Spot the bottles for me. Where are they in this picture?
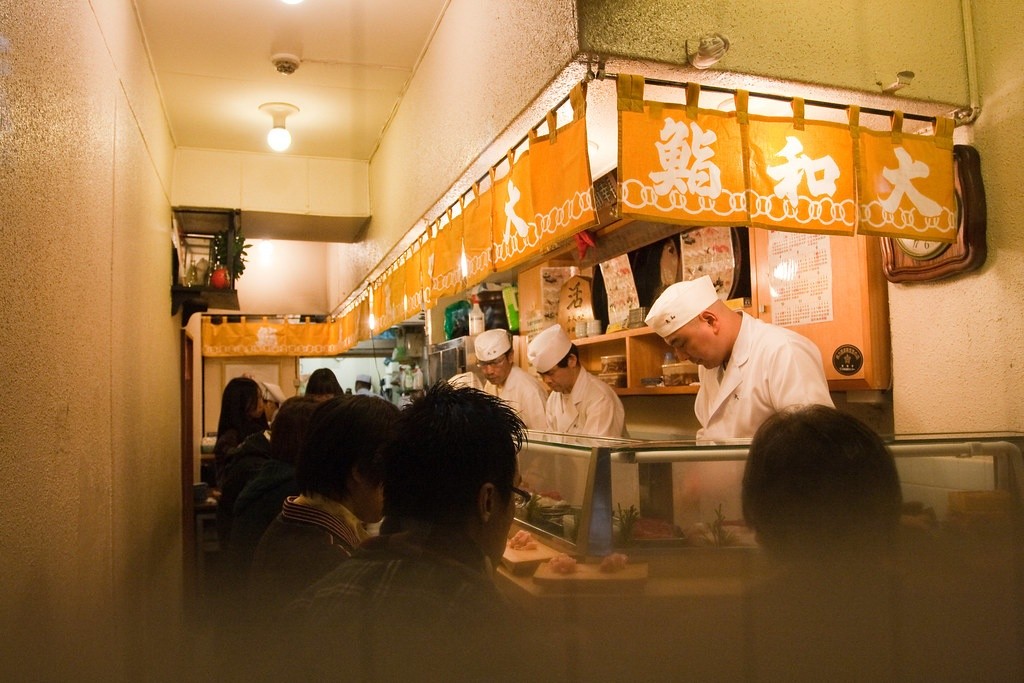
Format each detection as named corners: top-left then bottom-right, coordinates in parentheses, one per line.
top-left (664, 352), bottom-right (675, 364)
top-left (469, 299), bottom-right (485, 335)
top-left (400, 365), bottom-right (423, 389)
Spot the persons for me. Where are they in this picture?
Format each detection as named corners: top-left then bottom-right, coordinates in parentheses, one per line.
top-left (528, 326), bottom-right (638, 515)
top-left (470, 326), bottom-right (546, 430)
top-left (723, 405), bottom-right (905, 550)
top-left (644, 281), bottom-right (832, 536)
top-left (206, 369), bottom-right (530, 637)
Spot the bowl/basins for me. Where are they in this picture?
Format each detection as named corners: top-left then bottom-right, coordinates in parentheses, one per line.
top-left (575, 320), bottom-right (602, 338)
top-left (597, 373), bottom-right (627, 388)
top-left (600, 355), bottom-right (627, 373)
top-left (661, 363), bottom-right (699, 386)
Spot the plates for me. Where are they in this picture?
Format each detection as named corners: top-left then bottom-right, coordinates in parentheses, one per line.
top-left (627, 526), bottom-right (689, 542)
top-left (533, 562), bottom-right (649, 582)
top-left (501, 539), bottom-right (559, 573)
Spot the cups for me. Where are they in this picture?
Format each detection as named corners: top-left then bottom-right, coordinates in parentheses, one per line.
top-left (193, 482), bottom-right (208, 504)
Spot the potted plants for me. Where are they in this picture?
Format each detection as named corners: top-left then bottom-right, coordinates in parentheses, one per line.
top-left (209, 227), bottom-right (251, 290)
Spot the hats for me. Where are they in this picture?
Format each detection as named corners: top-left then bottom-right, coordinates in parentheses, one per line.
top-left (474, 329), bottom-right (511, 363)
top-left (356, 374), bottom-right (372, 383)
top-left (527, 323), bottom-right (572, 374)
top-left (644, 273), bottom-right (717, 337)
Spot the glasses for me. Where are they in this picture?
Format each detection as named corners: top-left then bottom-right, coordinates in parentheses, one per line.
top-left (474, 354), bottom-right (507, 368)
top-left (481, 480), bottom-right (532, 508)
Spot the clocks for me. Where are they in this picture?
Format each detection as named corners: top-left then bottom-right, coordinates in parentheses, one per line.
top-left (893, 186), bottom-right (962, 262)
